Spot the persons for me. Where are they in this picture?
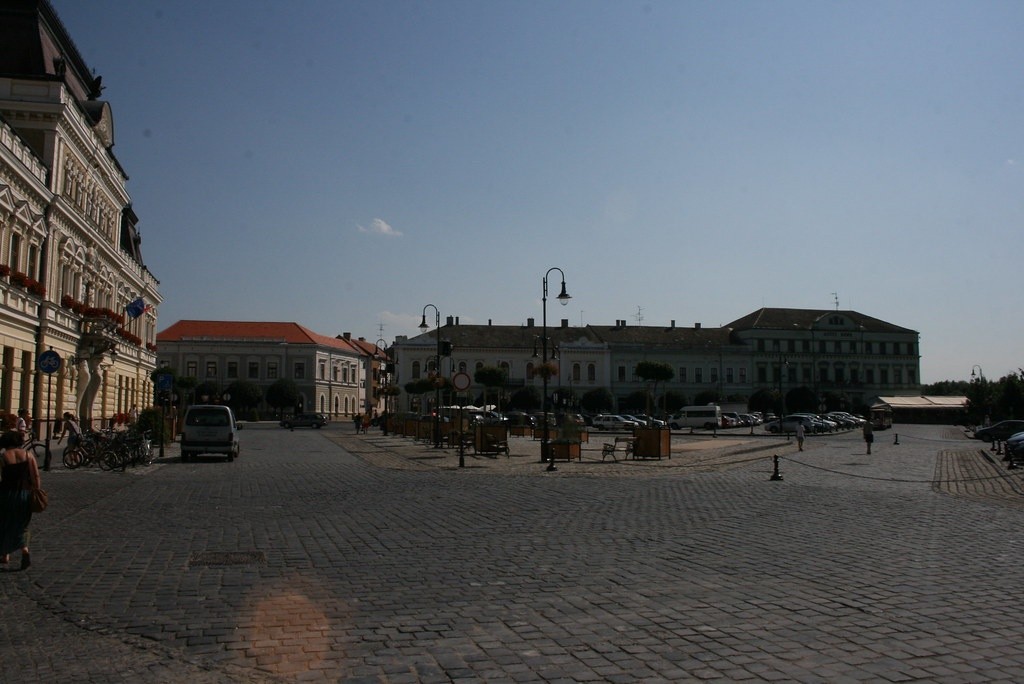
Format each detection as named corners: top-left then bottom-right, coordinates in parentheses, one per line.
top-left (362, 412), bottom-right (369, 434)
top-left (129, 404), bottom-right (138, 424)
top-left (863, 417), bottom-right (873, 454)
top-left (985, 415), bottom-right (990, 427)
top-left (0, 409), bottom-right (32, 453)
top-left (796, 421), bottom-right (805, 451)
top-left (374, 410), bottom-right (378, 419)
top-left (0, 430), bottom-right (40, 565)
top-left (57, 412), bottom-right (80, 466)
top-left (354, 413), bottom-right (361, 434)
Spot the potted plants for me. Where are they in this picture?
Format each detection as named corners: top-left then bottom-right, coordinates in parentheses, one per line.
top-left (630, 359), bottom-right (676, 462)
top-left (382, 365), bottom-right (590, 462)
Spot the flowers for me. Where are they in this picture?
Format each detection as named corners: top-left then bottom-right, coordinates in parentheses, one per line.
top-left (62, 294), bottom-right (159, 352)
top-left (0, 262), bottom-right (45, 295)
top-left (112, 412), bottom-right (131, 425)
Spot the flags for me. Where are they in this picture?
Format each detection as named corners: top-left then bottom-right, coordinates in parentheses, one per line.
top-left (126, 299), bottom-right (144, 318)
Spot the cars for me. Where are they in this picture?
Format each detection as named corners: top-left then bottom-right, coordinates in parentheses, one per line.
top-left (974, 420), bottom-right (1024, 461)
top-left (429, 403), bottom-right (874, 435)
top-left (280, 411), bottom-right (328, 429)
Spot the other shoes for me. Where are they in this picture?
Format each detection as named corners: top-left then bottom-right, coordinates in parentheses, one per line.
top-left (21, 551), bottom-right (31, 570)
top-left (800, 448), bottom-right (803, 451)
top-left (867, 450), bottom-right (871, 454)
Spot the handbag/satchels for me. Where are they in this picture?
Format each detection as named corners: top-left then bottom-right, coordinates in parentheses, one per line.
top-left (24, 486), bottom-right (48, 514)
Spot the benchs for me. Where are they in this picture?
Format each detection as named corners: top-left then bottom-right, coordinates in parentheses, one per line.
top-left (600, 434), bottom-right (641, 462)
top-left (484, 430), bottom-right (511, 460)
top-left (454, 430), bottom-right (474, 451)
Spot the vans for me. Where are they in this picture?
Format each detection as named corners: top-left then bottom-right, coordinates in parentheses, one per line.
top-left (667, 405), bottom-right (723, 430)
top-left (180, 404), bottom-right (243, 463)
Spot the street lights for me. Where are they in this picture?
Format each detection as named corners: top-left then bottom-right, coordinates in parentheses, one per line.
top-left (971, 364), bottom-right (986, 427)
top-left (373, 339), bottom-right (399, 437)
top-left (532, 267), bottom-right (573, 472)
top-left (793, 323), bottom-right (818, 413)
top-left (417, 304), bottom-right (456, 452)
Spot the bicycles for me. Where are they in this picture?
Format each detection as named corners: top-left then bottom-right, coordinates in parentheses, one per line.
top-left (17, 424), bottom-right (155, 472)
